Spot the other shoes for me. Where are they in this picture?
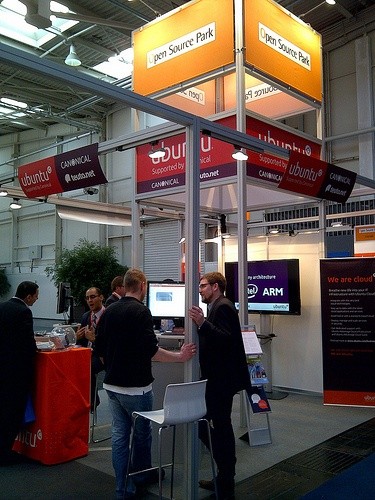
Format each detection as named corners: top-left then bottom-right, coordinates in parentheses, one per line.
top-left (91, 399), bottom-right (100, 411)
top-left (203, 493), bottom-right (218, 500)
top-left (130, 487), bottom-right (148, 500)
top-left (199, 479), bottom-right (215, 491)
top-left (0, 451), bottom-right (29, 465)
top-left (136, 469), bottom-right (165, 487)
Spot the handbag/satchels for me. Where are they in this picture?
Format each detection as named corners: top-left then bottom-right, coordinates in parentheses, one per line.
top-left (23, 395), bottom-right (35, 426)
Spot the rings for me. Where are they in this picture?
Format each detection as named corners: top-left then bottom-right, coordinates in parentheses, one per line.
top-left (192, 350), bottom-right (194, 353)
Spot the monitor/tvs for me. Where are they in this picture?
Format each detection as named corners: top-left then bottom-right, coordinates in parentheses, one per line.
top-left (147, 281), bottom-right (209, 318)
top-left (223, 260), bottom-right (301, 315)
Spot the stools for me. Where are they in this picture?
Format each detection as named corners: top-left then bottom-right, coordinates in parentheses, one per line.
top-left (122, 379), bottom-right (218, 500)
top-left (92, 373), bottom-right (111, 444)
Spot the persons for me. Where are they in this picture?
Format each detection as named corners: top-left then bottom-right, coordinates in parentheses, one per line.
top-left (94, 268), bottom-right (198, 500)
top-left (0, 281), bottom-right (38, 461)
top-left (75, 287), bottom-right (107, 411)
top-left (189, 272), bottom-right (252, 500)
top-left (106, 276), bottom-right (126, 308)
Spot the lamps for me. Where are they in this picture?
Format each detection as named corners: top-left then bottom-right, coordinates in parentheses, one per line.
top-left (10, 197), bottom-right (22, 209)
top-left (232, 143), bottom-right (249, 161)
top-left (65, 43), bottom-right (82, 67)
top-left (148, 141), bottom-right (166, 158)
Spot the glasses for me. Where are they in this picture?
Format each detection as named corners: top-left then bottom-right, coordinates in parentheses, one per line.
top-left (199, 282), bottom-right (221, 288)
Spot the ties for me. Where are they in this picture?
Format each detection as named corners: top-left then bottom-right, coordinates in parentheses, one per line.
top-left (91, 314), bottom-right (97, 329)
